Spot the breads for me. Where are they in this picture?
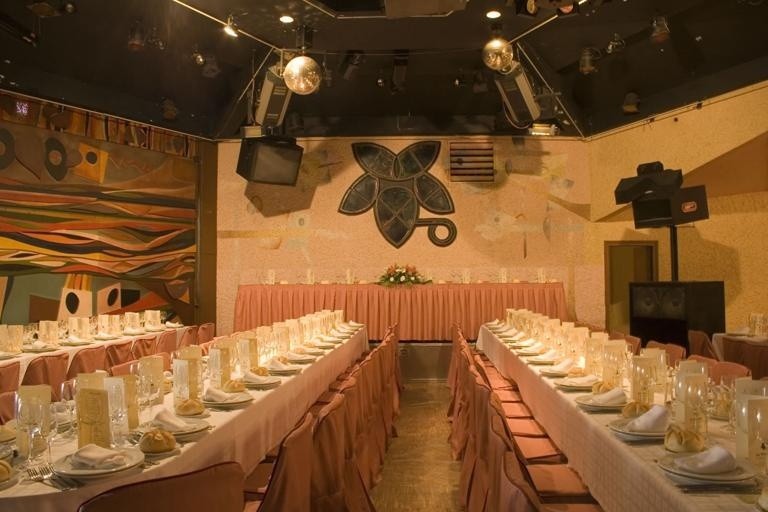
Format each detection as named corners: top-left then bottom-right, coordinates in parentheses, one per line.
top-left (304, 342), bottom-right (316, 348)
top-left (277, 356), bottom-right (288, 365)
top-left (1, 460), bottom-right (12, 482)
top-left (518, 335), bottom-right (704, 453)
top-left (294, 347), bottom-right (306, 355)
top-left (140, 428), bottom-right (176, 454)
top-left (223, 380), bottom-right (245, 392)
top-left (175, 397), bottom-right (204, 415)
top-left (250, 366), bottom-right (269, 376)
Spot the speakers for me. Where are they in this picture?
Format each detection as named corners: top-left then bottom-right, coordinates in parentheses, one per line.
top-left (629, 281), bottom-right (726, 358)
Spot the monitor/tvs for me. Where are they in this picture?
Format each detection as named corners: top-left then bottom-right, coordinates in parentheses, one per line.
top-left (236, 137), bottom-right (304, 186)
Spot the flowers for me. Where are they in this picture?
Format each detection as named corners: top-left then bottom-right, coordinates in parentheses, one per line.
top-left (375, 264), bottom-right (432, 283)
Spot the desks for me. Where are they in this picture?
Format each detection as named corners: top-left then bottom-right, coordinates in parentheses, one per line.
top-left (234, 283), bottom-right (568, 342)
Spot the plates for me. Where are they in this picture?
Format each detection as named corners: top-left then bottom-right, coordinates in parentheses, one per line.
top-left (0, 322), bottom-right (364, 493)
top-left (481, 319), bottom-right (756, 509)
